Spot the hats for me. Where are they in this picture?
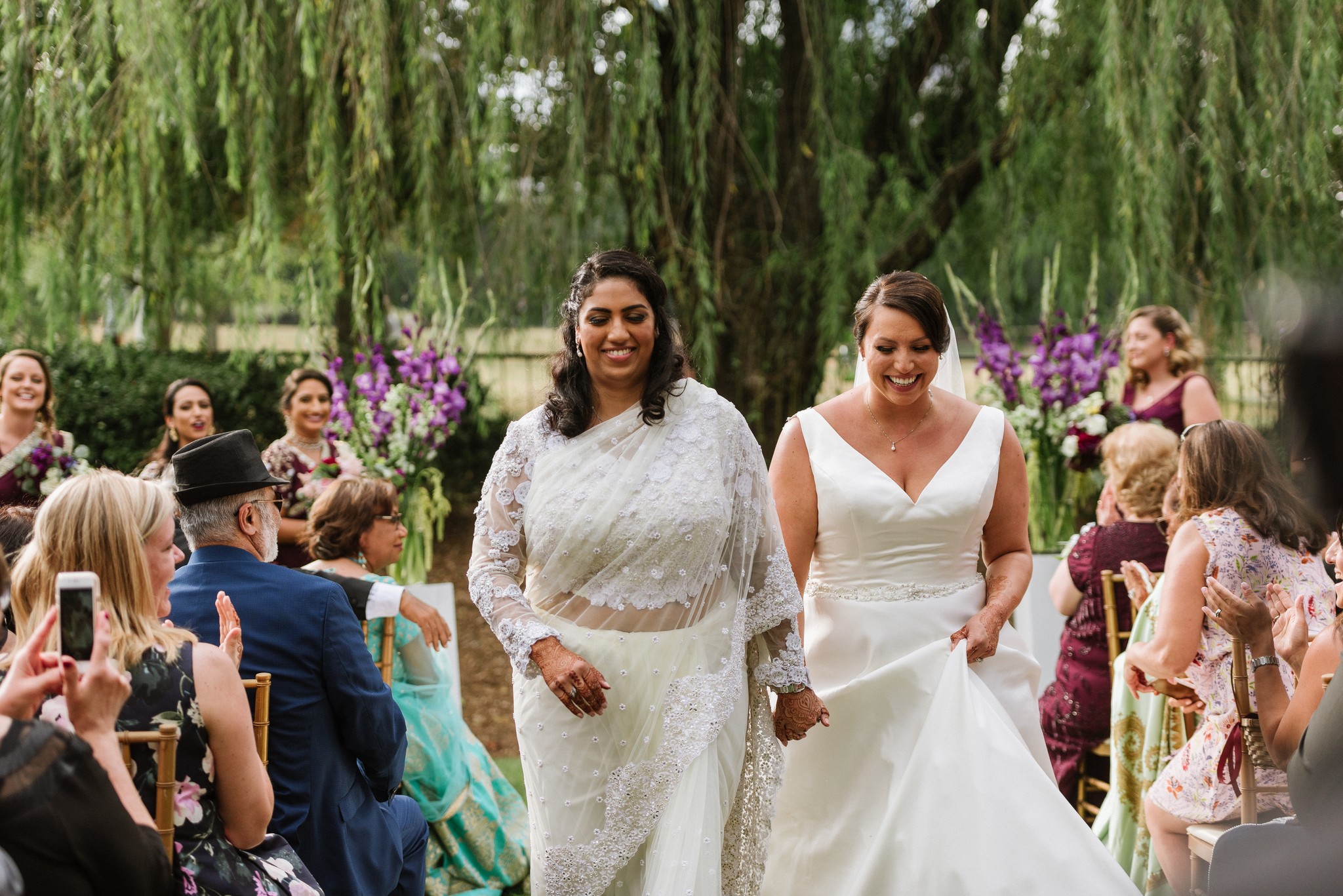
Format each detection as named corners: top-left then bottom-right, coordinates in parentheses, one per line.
top-left (173, 429), bottom-right (291, 501)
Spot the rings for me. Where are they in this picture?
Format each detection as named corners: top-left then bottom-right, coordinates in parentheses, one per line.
top-left (976, 658), bottom-right (984, 663)
top-left (568, 686), bottom-right (576, 699)
top-left (1214, 609), bottom-right (1221, 618)
top-left (1126, 588), bottom-right (1135, 600)
top-left (1273, 615), bottom-right (1280, 622)
top-left (816, 718), bottom-right (821, 723)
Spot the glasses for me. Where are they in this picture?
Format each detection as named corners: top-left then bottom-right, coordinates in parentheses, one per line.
top-left (1181, 422), bottom-right (1205, 441)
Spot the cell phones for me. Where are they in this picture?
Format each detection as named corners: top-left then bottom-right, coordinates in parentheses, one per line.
top-left (47, 568), bottom-right (101, 676)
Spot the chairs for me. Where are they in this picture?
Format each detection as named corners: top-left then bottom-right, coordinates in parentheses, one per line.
top-left (114, 613), bottom-right (395, 865)
top-left (1075, 567), bottom-right (1309, 896)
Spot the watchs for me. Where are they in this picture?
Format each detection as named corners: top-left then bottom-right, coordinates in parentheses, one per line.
top-left (767, 679), bottom-right (805, 694)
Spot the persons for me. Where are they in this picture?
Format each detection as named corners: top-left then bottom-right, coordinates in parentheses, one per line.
top-left (1118, 302), bottom-right (1225, 439)
top-left (260, 365), bottom-right (373, 572)
top-left (0, 346), bottom-right (88, 505)
top-left (1120, 421), bottom-right (1339, 896)
top-left (295, 472), bottom-right (533, 896)
top-left (0, 600), bottom-right (176, 895)
top-left (8, 462), bottom-right (328, 896)
top-left (463, 247), bottom-right (832, 896)
top-left (765, 269), bottom-right (1151, 896)
top-left (152, 375), bottom-right (217, 465)
top-left (1032, 418), bottom-right (1183, 808)
top-left (134, 425), bottom-right (434, 896)
top-left (1081, 471), bottom-right (1206, 896)
top-left (1199, 513), bottom-right (1343, 774)
top-left (1, 505), bottom-right (40, 631)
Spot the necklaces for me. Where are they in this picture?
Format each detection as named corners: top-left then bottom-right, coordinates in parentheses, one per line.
top-left (591, 405), bottom-right (628, 440)
top-left (865, 383), bottom-right (933, 451)
top-left (283, 438), bottom-right (326, 450)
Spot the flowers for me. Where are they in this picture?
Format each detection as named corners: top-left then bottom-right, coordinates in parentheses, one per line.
top-left (944, 235), bottom-right (1141, 555)
top-left (296, 258), bottom-right (500, 582)
top-left (17, 433), bottom-right (92, 502)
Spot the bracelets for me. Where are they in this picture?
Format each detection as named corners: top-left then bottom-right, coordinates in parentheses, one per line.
top-left (1249, 655), bottom-right (1280, 673)
